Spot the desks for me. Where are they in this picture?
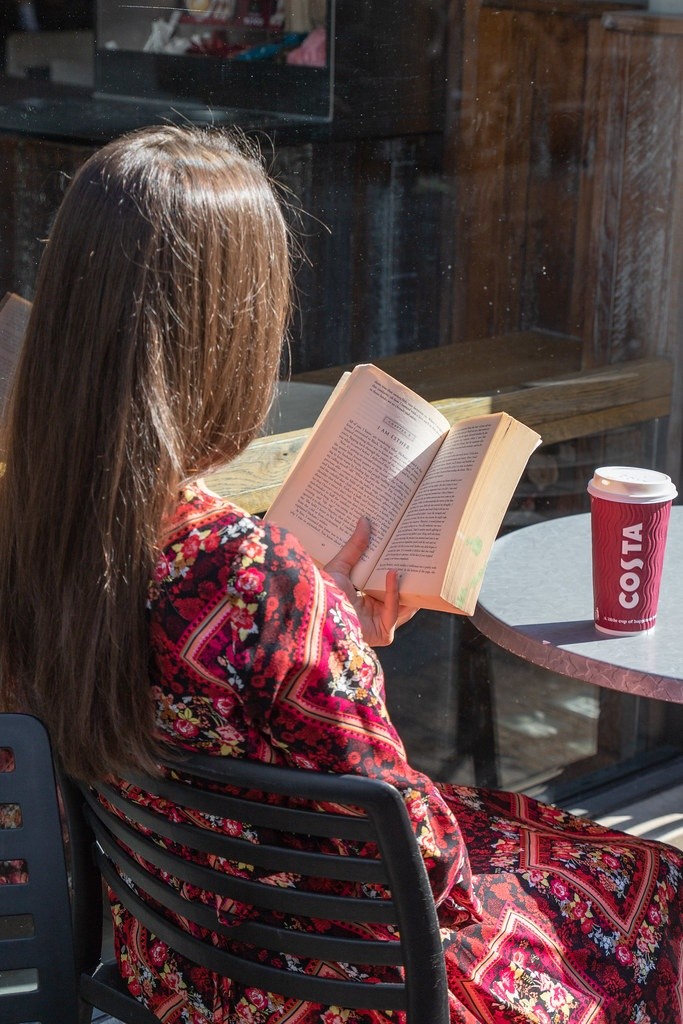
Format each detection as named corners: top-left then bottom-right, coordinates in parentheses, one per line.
top-left (194, 328), bottom-right (671, 757)
top-left (466, 504), bottom-right (683, 704)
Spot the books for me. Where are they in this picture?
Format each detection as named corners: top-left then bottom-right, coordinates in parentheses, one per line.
top-left (264, 363), bottom-right (540, 616)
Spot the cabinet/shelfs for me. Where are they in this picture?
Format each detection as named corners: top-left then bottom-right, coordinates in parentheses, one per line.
top-left (0, 75), bottom-right (442, 380)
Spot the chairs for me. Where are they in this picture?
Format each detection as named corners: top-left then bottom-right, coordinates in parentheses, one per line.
top-left (0, 712), bottom-right (452, 1024)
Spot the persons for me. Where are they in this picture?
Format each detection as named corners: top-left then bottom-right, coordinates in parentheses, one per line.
top-left (1, 119), bottom-right (683, 1024)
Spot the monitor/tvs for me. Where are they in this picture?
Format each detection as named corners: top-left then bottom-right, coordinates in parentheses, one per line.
top-left (94, 1), bottom-right (335, 124)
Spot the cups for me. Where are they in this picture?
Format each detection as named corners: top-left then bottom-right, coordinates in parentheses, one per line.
top-left (587, 463), bottom-right (678, 636)
